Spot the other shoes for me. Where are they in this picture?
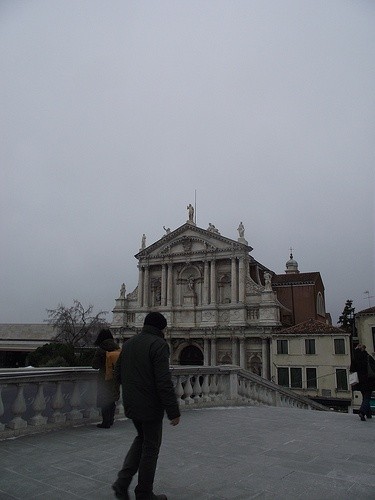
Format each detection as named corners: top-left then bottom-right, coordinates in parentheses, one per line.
top-left (366, 413), bottom-right (372, 419)
top-left (136, 492), bottom-right (167, 500)
top-left (97, 423), bottom-right (113, 428)
top-left (359, 412), bottom-right (366, 421)
top-left (112, 480), bottom-right (129, 500)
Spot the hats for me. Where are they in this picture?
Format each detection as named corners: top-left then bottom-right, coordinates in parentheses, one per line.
top-left (144, 312), bottom-right (167, 330)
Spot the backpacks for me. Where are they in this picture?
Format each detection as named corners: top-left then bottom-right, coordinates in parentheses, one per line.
top-left (105, 349), bottom-right (122, 381)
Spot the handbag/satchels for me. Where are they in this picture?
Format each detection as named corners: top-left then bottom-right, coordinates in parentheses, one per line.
top-left (349, 372), bottom-right (359, 386)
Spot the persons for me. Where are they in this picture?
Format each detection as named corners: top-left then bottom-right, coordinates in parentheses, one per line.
top-left (120, 284), bottom-right (126, 298)
top-left (264, 271), bottom-right (272, 291)
top-left (187, 204), bottom-right (194, 221)
top-left (92, 330), bottom-right (120, 428)
top-left (109, 312), bottom-right (181, 500)
top-left (237, 223), bottom-right (245, 237)
top-left (350, 344), bottom-right (375, 421)
top-left (141, 233), bottom-right (146, 248)
top-left (163, 226), bottom-right (171, 235)
top-left (207, 223), bottom-right (218, 232)
